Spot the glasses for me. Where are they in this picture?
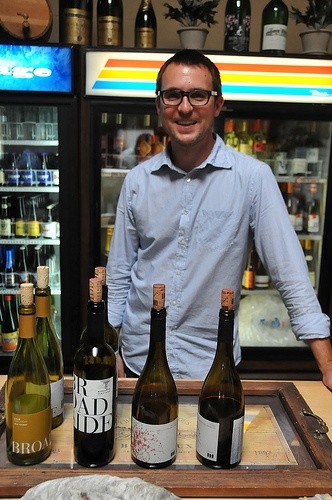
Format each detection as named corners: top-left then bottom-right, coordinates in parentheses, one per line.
top-left (157, 86), bottom-right (219, 106)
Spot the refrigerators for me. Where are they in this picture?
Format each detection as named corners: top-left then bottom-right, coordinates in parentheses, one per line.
top-left (0, 42), bottom-right (332, 382)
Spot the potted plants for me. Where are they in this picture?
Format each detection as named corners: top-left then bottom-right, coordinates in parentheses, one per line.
top-left (289, 0), bottom-right (332, 54)
top-left (162, 0), bottom-right (220, 51)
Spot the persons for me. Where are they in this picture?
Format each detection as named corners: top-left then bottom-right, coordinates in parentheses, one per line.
top-left (106, 50), bottom-right (332, 390)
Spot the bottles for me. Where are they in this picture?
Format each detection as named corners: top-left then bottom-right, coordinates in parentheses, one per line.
top-left (259, 0), bottom-right (289, 54)
top-left (6, 283), bottom-right (52, 466)
top-left (34, 265), bottom-right (64, 430)
top-left (223, 0), bottom-right (251, 51)
top-left (101, 110), bottom-right (321, 289)
top-left (58, 0), bottom-right (93, 46)
top-left (96, 0), bottom-right (124, 47)
top-left (0, 149), bottom-right (61, 353)
top-left (71, 279), bottom-right (118, 468)
top-left (130, 284), bottom-right (179, 469)
top-left (93, 267), bottom-right (119, 365)
top-left (134, 0), bottom-right (157, 49)
top-left (195, 288), bottom-right (245, 469)
